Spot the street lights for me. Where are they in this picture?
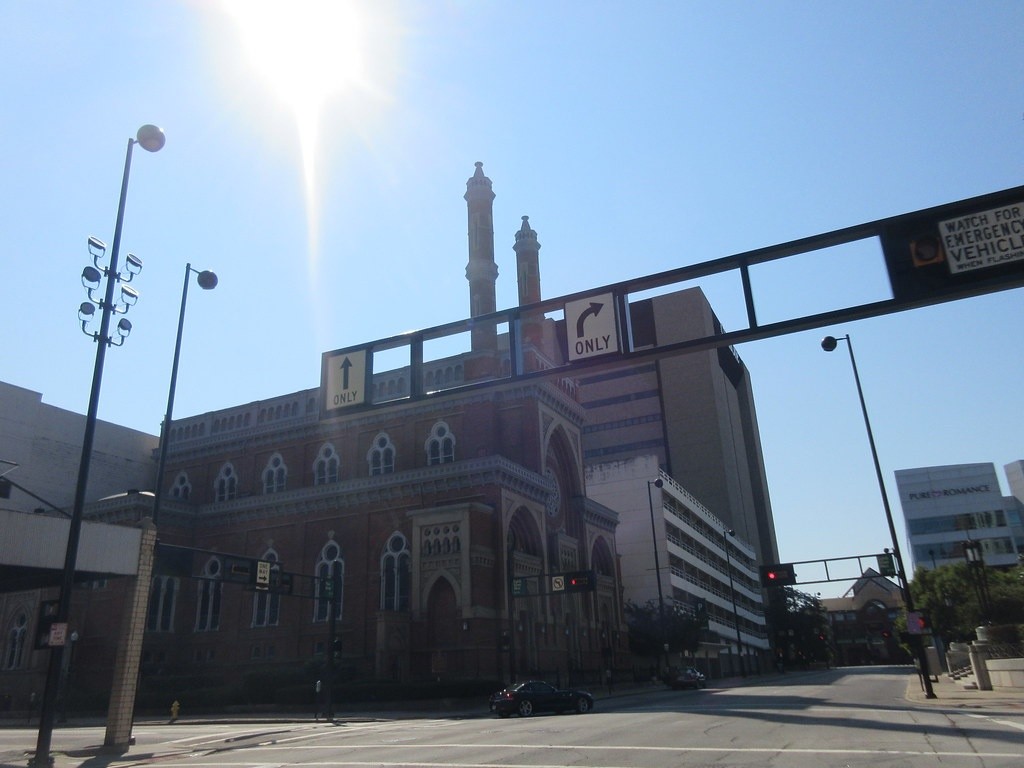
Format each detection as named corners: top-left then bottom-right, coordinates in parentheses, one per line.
top-left (98, 259), bottom-right (222, 753)
top-left (647, 478), bottom-right (671, 688)
top-left (823, 330), bottom-right (938, 699)
top-left (25, 124), bottom-right (167, 768)
top-left (722, 528), bottom-right (749, 676)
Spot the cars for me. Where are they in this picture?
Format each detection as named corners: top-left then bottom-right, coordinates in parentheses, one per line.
top-left (676, 667), bottom-right (705, 689)
top-left (488, 678), bottom-right (595, 719)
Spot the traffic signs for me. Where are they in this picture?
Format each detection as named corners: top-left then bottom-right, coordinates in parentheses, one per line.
top-left (325, 350), bottom-right (364, 409)
top-left (563, 292), bottom-right (619, 363)
top-left (256, 561), bottom-right (270, 592)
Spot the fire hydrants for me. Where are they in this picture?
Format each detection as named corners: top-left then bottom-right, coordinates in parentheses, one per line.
top-left (170, 700), bottom-right (180, 719)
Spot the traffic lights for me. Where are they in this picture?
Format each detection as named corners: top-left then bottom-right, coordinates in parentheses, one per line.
top-left (759, 564), bottom-right (796, 586)
top-left (565, 575), bottom-right (576, 590)
top-left (915, 612), bottom-right (932, 631)
